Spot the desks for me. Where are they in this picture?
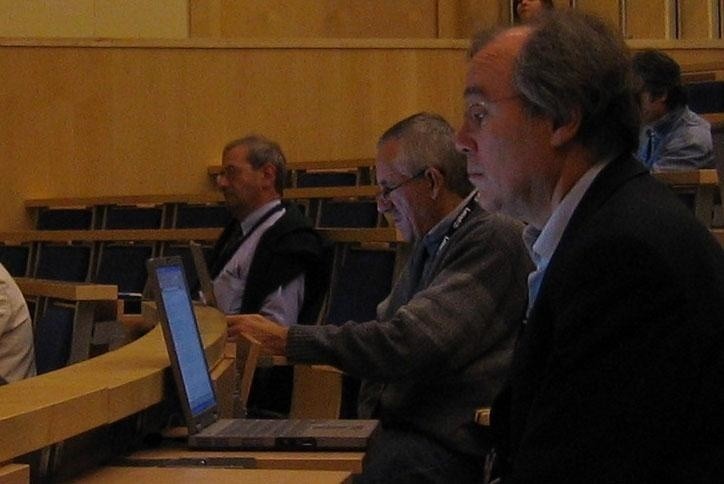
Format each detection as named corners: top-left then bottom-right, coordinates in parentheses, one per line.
top-left (0, 81), bottom-right (724, 484)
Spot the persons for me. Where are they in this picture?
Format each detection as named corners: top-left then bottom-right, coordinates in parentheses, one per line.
top-left (222, 110), bottom-right (536, 481)
top-left (0, 263), bottom-right (37, 385)
top-left (192, 132), bottom-right (335, 420)
top-left (515, 0), bottom-right (552, 26)
top-left (450, 6), bottom-right (724, 483)
top-left (625, 44), bottom-right (722, 220)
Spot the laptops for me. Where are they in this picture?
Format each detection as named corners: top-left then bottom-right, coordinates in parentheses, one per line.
top-left (187, 237), bottom-right (219, 310)
top-left (148, 253), bottom-right (384, 448)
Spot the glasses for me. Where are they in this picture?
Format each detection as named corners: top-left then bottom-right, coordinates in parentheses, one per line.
top-left (460, 95), bottom-right (521, 126)
top-left (372, 168), bottom-right (427, 203)
